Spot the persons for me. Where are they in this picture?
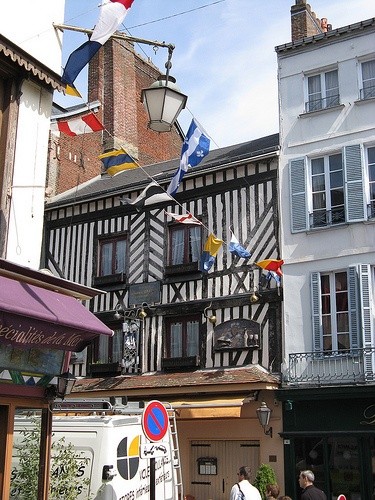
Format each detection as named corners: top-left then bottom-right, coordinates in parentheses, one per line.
top-left (297, 470), bottom-right (328, 500)
top-left (230, 465), bottom-right (262, 500)
top-left (266, 484), bottom-right (292, 500)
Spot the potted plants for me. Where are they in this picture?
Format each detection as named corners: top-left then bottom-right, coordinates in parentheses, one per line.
top-left (89, 360), bottom-right (122, 374)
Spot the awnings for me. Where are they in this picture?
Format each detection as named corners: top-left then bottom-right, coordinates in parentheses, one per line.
top-left (0, 275), bottom-right (114, 352)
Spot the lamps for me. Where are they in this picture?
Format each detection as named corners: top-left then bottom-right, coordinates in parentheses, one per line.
top-left (204, 301), bottom-right (217, 324)
top-left (51, 21), bottom-right (188, 134)
top-left (250, 286), bottom-right (263, 303)
top-left (113, 304), bottom-right (126, 321)
top-left (138, 301), bottom-right (154, 319)
top-left (255, 400), bottom-right (273, 438)
top-left (44, 368), bottom-right (77, 401)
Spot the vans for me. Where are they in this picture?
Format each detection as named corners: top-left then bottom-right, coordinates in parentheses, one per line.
top-left (10, 401), bottom-right (184, 500)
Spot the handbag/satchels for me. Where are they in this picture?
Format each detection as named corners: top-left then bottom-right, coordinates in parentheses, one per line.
top-left (235, 482), bottom-right (246, 500)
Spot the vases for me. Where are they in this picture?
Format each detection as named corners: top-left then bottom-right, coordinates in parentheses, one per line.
top-left (162, 354), bottom-right (201, 369)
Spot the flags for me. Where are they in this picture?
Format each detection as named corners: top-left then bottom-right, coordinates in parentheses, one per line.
top-left (58, 108), bottom-right (282, 297)
top-left (61, 0), bottom-right (134, 84)
top-left (62, 70), bottom-right (83, 97)
